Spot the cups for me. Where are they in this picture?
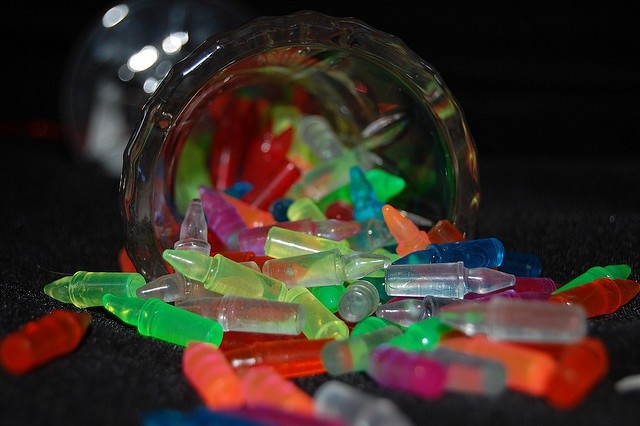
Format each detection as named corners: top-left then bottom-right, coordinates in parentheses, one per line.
top-left (120, 11), bottom-right (481, 282)
